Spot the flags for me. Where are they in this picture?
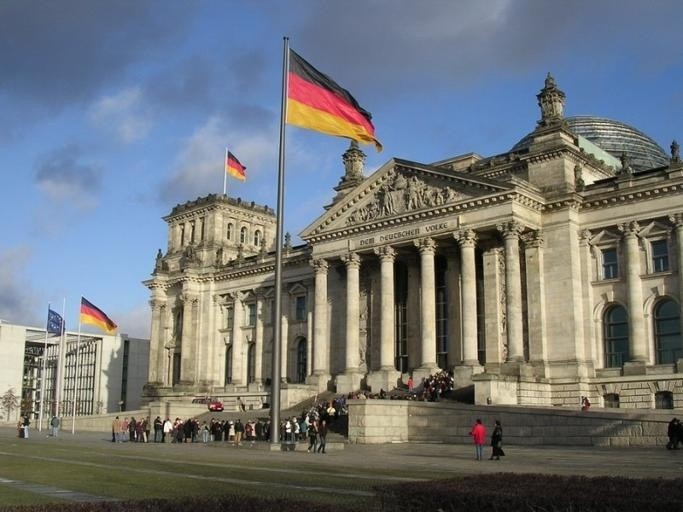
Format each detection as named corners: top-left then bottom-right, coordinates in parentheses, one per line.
top-left (47, 309), bottom-right (66, 337)
top-left (80, 296), bottom-right (118, 334)
top-left (286, 46), bottom-right (383, 153)
top-left (227, 150), bottom-right (248, 183)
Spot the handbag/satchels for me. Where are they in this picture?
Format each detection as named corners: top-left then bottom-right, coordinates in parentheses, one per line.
top-left (308, 430), bottom-right (316, 436)
top-left (494, 448), bottom-right (505, 456)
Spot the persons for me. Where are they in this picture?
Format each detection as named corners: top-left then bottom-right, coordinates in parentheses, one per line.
top-left (51, 416), bottom-right (60, 437)
top-left (582, 396), bottom-right (590, 410)
top-left (300, 366), bottom-right (455, 441)
top-left (111, 415), bottom-right (300, 446)
top-left (469, 418), bottom-right (486, 461)
top-left (19, 414), bottom-right (32, 427)
top-left (488, 420), bottom-right (503, 460)
top-left (318, 420), bottom-right (328, 454)
top-left (666, 418), bottom-right (683, 450)
top-left (308, 420), bottom-right (319, 453)
top-left (349, 173), bottom-right (456, 226)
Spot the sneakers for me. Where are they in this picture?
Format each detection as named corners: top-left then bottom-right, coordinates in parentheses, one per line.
top-left (488, 457), bottom-right (500, 460)
top-left (308, 448), bottom-right (325, 453)
